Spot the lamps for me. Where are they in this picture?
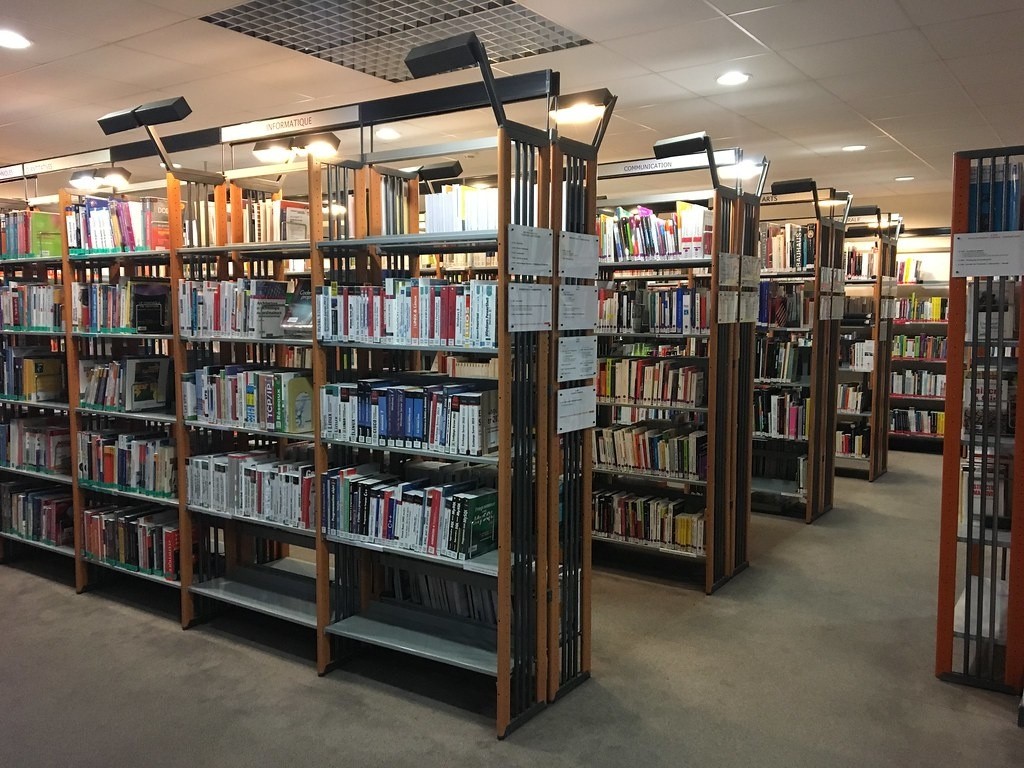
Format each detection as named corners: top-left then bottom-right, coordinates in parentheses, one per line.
top-left (402, 31), bottom-right (506, 125)
top-left (67, 167), bottom-right (132, 190)
top-left (550, 88), bottom-right (614, 124)
top-left (250, 131), bottom-right (341, 161)
top-left (95, 95), bottom-right (192, 137)
top-left (843, 204), bottom-right (882, 223)
top-left (398, 160), bottom-right (464, 182)
top-left (741, 155), bottom-right (772, 199)
top-left (652, 130), bottom-right (719, 190)
top-left (891, 213), bottom-right (899, 221)
top-left (834, 191), bottom-right (854, 224)
top-left (881, 212), bottom-right (891, 222)
top-left (771, 178), bottom-right (820, 220)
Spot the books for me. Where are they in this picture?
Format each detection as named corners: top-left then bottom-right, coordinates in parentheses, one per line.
top-left (0, 207), bottom-right (75, 551)
top-left (594, 161), bottom-right (1023, 560)
top-left (314, 183), bottom-right (585, 639)
top-left (175, 178), bottom-right (324, 563)
top-left (64, 192), bottom-right (205, 586)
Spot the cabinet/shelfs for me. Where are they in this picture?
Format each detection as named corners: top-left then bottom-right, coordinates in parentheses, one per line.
top-left (0, 69), bottom-right (1024, 740)
